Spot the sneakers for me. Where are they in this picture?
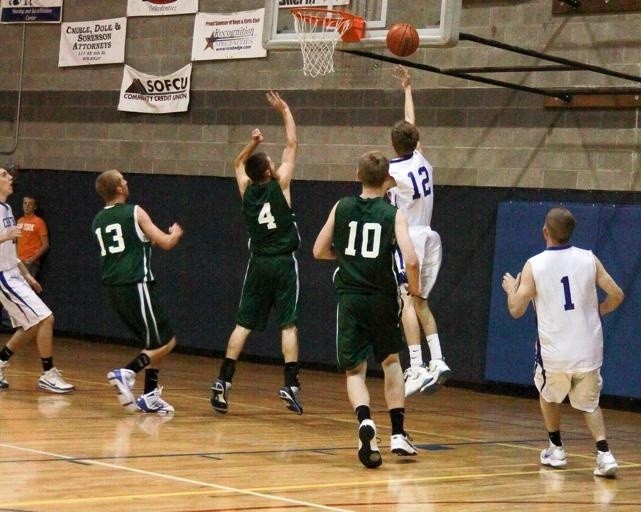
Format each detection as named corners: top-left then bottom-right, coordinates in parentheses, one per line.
top-left (107, 368), bottom-right (175, 414)
top-left (279, 385), bottom-right (304, 415)
top-left (404, 358), bottom-right (453, 400)
top-left (357, 419), bottom-right (382, 468)
top-left (539, 438), bottom-right (569, 468)
top-left (0, 358), bottom-right (9, 390)
top-left (37, 367), bottom-right (76, 394)
top-left (210, 378), bottom-right (232, 414)
top-left (390, 433), bottom-right (418, 457)
top-left (592, 449), bottom-right (618, 477)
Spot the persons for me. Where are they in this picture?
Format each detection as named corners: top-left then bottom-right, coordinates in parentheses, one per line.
top-left (14, 190), bottom-right (50, 278)
top-left (501, 208), bottom-right (625, 477)
top-left (0, 166), bottom-right (76, 394)
top-left (375, 63), bottom-right (453, 399)
top-left (210, 91), bottom-right (304, 418)
top-left (313, 150), bottom-right (419, 469)
top-left (91, 170), bottom-right (184, 414)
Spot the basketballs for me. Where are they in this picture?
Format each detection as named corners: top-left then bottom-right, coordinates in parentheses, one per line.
top-left (386, 23), bottom-right (418, 56)
